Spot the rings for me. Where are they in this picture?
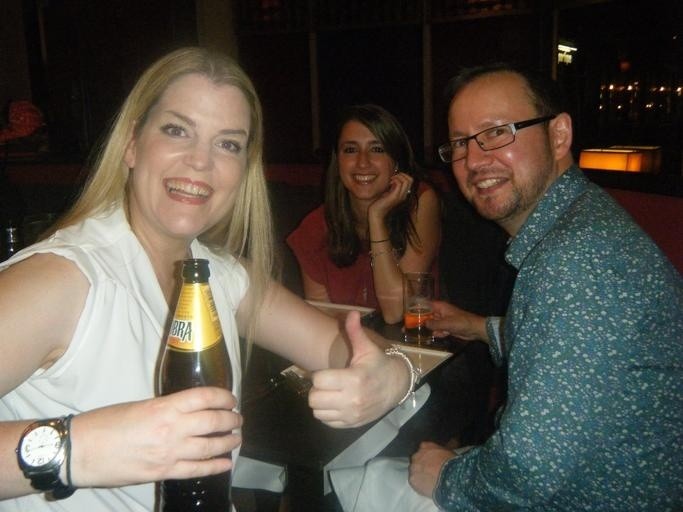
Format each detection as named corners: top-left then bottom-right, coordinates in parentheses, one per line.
top-left (406, 188), bottom-right (413, 195)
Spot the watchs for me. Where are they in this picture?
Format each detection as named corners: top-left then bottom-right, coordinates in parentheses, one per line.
top-left (14, 417), bottom-right (70, 503)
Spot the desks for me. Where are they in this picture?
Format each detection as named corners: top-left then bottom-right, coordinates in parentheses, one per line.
top-left (234, 301), bottom-right (496, 511)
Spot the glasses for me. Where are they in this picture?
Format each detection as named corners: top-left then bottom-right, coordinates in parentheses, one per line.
top-left (437, 114), bottom-right (557, 164)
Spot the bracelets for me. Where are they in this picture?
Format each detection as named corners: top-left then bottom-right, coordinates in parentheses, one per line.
top-left (367, 248), bottom-right (393, 272)
top-left (65, 413), bottom-right (74, 489)
top-left (383, 348), bottom-right (415, 408)
top-left (369, 238), bottom-right (388, 244)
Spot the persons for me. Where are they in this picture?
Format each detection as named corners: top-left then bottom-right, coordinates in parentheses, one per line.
top-left (0, 98), bottom-right (76, 221)
top-left (285, 99), bottom-right (445, 458)
top-left (0, 44), bottom-right (418, 512)
top-left (327, 53), bottom-right (681, 512)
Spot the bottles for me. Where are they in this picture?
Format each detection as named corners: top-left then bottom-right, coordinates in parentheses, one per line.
top-left (5, 218), bottom-right (23, 261)
top-left (158, 258), bottom-right (233, 512)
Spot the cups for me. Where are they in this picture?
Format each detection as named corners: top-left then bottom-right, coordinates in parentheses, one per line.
top-left (404, 275), bottom-right (435, 346)
